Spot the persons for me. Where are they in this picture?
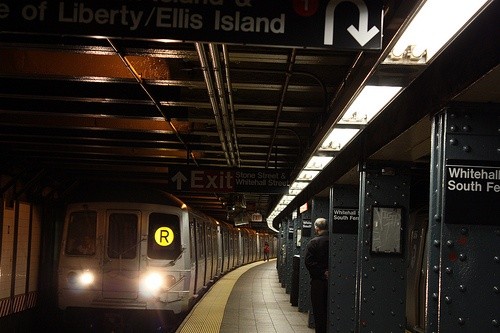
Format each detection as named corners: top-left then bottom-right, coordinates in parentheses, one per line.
top-left (78, 234), bottom-right (96, 256)
top-left (264, 242), bottom-right (270, 262)
top-left (304, 217), bottom-right (329, 333)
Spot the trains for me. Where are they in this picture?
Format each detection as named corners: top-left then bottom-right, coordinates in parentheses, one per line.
top-left (58, 188), bottom-right (277, 324)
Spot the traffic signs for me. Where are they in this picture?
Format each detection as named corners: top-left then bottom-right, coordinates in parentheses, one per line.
top-left (0, 0), bottom-right (386, 51)
